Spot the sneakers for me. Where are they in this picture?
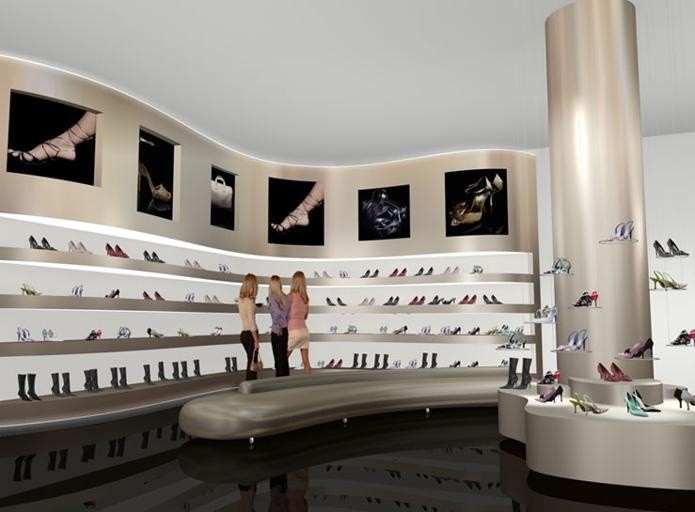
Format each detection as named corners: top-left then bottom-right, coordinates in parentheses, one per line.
top-left (666, 329), bottom-right (691, 346)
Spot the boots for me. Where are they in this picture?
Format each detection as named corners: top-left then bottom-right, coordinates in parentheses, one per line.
top-left (143, 364), bottom-right (151, 384)
top-left (83, 369), bottom-right (98, 392)
top-left (157, 361), bottom-right (166, 382)
top-left (172, 362), bottom-right (180, 380)
top-left (193, 360), bottom-right (201, 377)
top-left (61, 372), bottom-right (72, 396)
top-left (51, 373), bottom-right (61, 397)
top-left (17, 373), bottom-right (32, 402)
top-left (430, 353), bottom-right (437, 368)
top-left (110, 368), bottom-right (119, 389)
top-left (27, 373), bottom-right (42, 401)
top-left (181, 361), bottom-right (188, 378)
top-left (513, 358), bottom-right (532, 390)
top-left (231, 356), bottom-right (237, 372)
top-left (499, 357), bottom-right (519, 389)
top-left (119, 367), bottom-right (128, 387)
top-left (419, 352), bottom-right (428, 368)
top-left (225, 357), bottom-right (231, 373)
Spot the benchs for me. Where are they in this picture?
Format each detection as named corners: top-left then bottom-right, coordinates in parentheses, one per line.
top-left (179, 365), bottom-right (512, 446)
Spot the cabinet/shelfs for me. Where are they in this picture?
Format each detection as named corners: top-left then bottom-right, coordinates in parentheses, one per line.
top-left (498, 377), bottom-right (695, 491)
top-left (0, 245), bottom-right (543, 434)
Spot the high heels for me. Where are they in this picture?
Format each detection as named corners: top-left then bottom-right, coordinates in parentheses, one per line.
top-left (334, 359), bottom-right (343, 367)
top-left (21, 282), bottom-right (42, 296)
top-left (42, 328), bottom-right (53, 341)
top-left (616, 221), bottom-right (634, 241)
top-left (115, 244), bottom-right (129, 258)
top-left (104, 288), bottom-right (120, 299)
top-left (398, 268), bottom-right (407, 276)
top-left (216, 262), bottom-right (232, 273)
top-left (212, 295), bottom-right (224, 304)
top-left (389, 268), bottom-right (399, 277)
top-left (360, 269), bottom-right (370, 278)
top-left (326, 359), bottom-right (335, 367)
top-left (449, 361), bottom-right (461, 368)
top-left (351, 353), bottom-right (359, 368)
top-left (105, 243), bottom-right (118, 256)
top-left (468, 326), bottom-right (480, 335)
top-left (183, 257), bottom-right (193, 268)
top-left (184, 292), bottom-right (195, 303)
top-left (338, 270), bottom-right (349, 278)
top-left (603, 223), bottom-right (625, 241)
top-left (624, 392), bottom-right (648, 417)
top-left (359, 298), bottom-right (368, 305)
top-left (68, 240), bottom-right (82, 253)
top-left (211, 327), bottom-right (224, 335)
top-left (368, 298), bottom-right (376, 305)
top-left (552, 371), bottom-right (560, 384)
top-left (392, 325), bottom-right (408, 334)
top-left (147, 328), bottom-right (163, 338)
top-left (564, 328), bottom-right (587, 352)
top-left (542, 257), bottom-right (571, 274)
top-left (608, 361), bottom-right (632, 381)
top-left (143, 250), bottom-right (154, 261)
top-left (589, 290), bottom-right (598, 307)
top-left (407, 360), bottom-right (417, 369)
top-left (177, 328), bottom-right (189, 337)
top-left (322, 270), bottom-right (332, 278)
top-left (152, 251), bottom-right (166, 264)
top-left (193, 261), bottom-right (203, 270)
top-left (117, 326), bottom-right (131, 340)
top-left (154, 291), bottom-right (164, 300)
top-left (485, 328), bottom-right (498, 335)
top-left (70, 285), bottom-right (84, 297)
top-left (87, 329), bottom-right (97, 339)
top-left (41, 237), bottom-right (57, 250)
top-left (138, 137), bottom-right (171, 201)
top-left (96, 328), bottom-right (102, 339)
top-left (7, 134), bottom-right (76, 173)
top-left (313, 271), bottom-right (322, 278)
top-left (419, 326), bottom-right (430, 336)
top-left (491, 295), bottom-right (503, 304)
top-left (659, 272), bottom-right (687, 291)
top-left (471, 265), bottom-right (483, 274)
top-left (344, 325), bottom-right (358, 335)
top-left (204, 294), bottom-right (212, 303)
top-left (439, 327), bottom-right (449, 335)
top-left (443, 266), bottom-right (451, 273)
top-left (383, 296), bottom-right (394, 305)
top-left (569, 392), bottom-right (591, 414)
top-left (381, 354), bottom-right (389, 369)
top-left (326, 297), bottom-right (336, 306)
top-left (496, 327), bottom-right (528, 349)
top-left (28, 235), bottom-right (45, 249)
top-left (337, 297), bottom-right (347, 306)
top-left (545, 385), bottom-right (563, 403)
top-left (580, 394), bottom-right (608, 416)
top-left (482, 295), bottom-right (493, 304)
top-left (494, 324), bottom-right (508, 334)
top-left (632, 338), bottom-right (654, 358)
top-left (143, 291), bottom-right (152, 300)
top-left (270, 213), bottom-right (311, 238)
top-left (674, 388), bottom-right (683, 408)
top-left (618, 338), bottom-right (645, 359)
top-left (650, 270), bottom-right (670, 289)
top-left (428, 295), bottom-right (444, 304)
top-left (467, 361), bottom-right (478, 368)
top-left (369, 269), bottom-right (379, 277)
top-left (531, 305), bottom-right (560, 324)
top-left (555, 330), bottom-right (578, 350)
top-left (450, 327), bottom-right (461, 335)
top-left (360, 353), bottom-right (367, 368)
top-left (443, 298), bottom-right (456, 304)
top-left (16, 328), bottom-right (32, 342)
top-left (408, 296), bottom-right (418, 305)
top-left (653, 240), bottom-right (674, 258)
top-left (452, 266), bottom-right (460, 273)
top-left (372, 353), bottom-right (380, 368)
top-left (538, 370), bottom-right (553, 384)
top-left (596, 363), bottom-right (619, 382)
top-left (498, 360), bottom-right (508, 367)
top-left (390, 296), bottom-right (399, 305)
top-left (534, 386), bottom-right (556, 404)
top-left (681, 389), bottom-right (695, 411)
top-left (458, 294), bottom-right (469, 304)
top-left (450, 173), bottom-right (502, 229)
top-left (414, 267), bottom-right (424, 276)
top-left (632, 389), bottom-right (661, 413)
top-left (390, 360), bottom-right (401, 369)
top-left (574, 291), bottom-right (592, 308)
top-left (416, 296), bottom-right (425, 304)
top-left (667, 239), bottom-right (689, 256)
top-left (467, 294), bottom-right (477, 304)
top-left (424, 267), bottom-right (433, 275)
top-left (77, 241), bottom-right (93, 254)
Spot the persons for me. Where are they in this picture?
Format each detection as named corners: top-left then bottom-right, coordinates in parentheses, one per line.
top-left (238, 274), bottom-right (260, 380)
top-left (271, 182), bottom-right (324, 233)
top-left (266, 275), bottom-right (290, 376)
top-left (287, 271), bottom-right (313, 374)
top-left (8, 111), bottom-right (96, 164)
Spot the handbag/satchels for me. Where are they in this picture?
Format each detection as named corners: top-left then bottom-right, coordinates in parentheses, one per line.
top-left (210, 175), bottom-right (233, 209)
top-left (250, 348), bottom-right (263, 371)
